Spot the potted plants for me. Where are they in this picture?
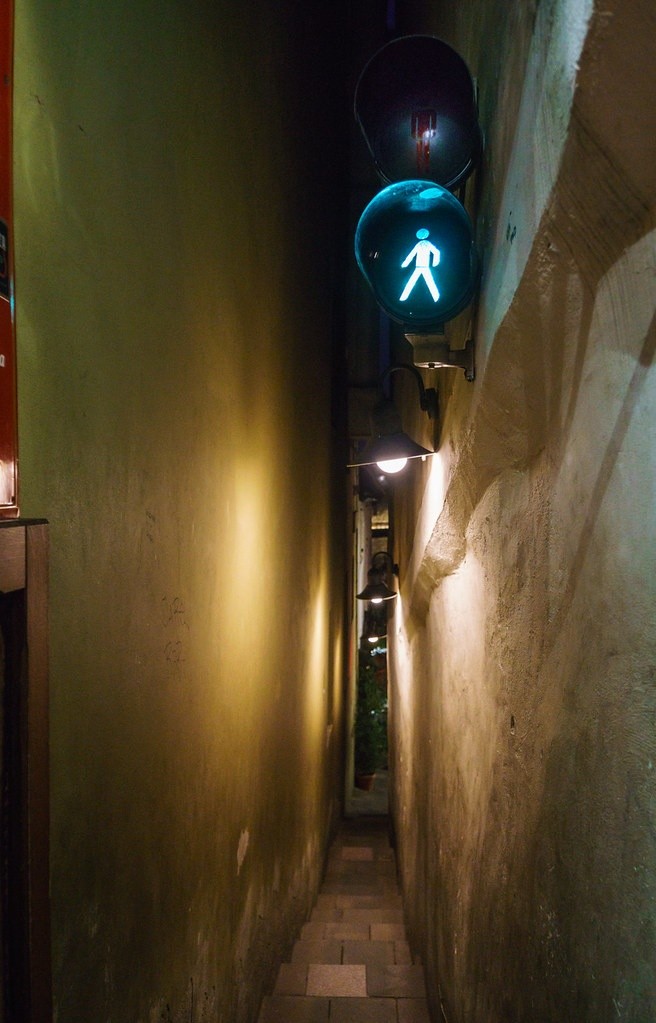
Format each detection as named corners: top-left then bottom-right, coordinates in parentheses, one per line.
top-left (355, 666), bottom-right (387, 790)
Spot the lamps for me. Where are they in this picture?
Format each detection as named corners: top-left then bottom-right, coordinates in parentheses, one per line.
top-left (346, 365), bottom-right (437, 476)
top-left (357, 552), bottom-right (401, 604)
top-left (360, 615), bottom-right (388, 643)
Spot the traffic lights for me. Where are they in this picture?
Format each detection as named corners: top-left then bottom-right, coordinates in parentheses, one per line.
top-left (349, 31), bottom-right (484, 334)
